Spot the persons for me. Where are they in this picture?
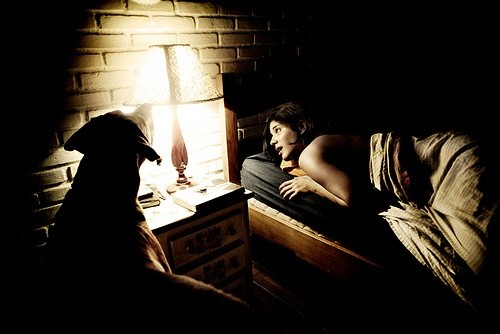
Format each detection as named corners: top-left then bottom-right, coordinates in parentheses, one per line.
top-left (266, 104), bottom-right (351, 207)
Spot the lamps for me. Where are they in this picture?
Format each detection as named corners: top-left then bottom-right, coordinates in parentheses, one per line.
top-left (125, 43), bottom-right (225, 193)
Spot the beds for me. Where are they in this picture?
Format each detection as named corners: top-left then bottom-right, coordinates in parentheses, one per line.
top-left (226, 106), bottom-right (499, 308)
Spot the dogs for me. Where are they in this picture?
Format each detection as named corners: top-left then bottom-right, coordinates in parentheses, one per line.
top-left (41, 103), bottom-right (173, 274)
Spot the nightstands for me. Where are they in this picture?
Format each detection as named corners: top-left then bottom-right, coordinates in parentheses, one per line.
top-left (137, 174), bottom-right (256, 312)
